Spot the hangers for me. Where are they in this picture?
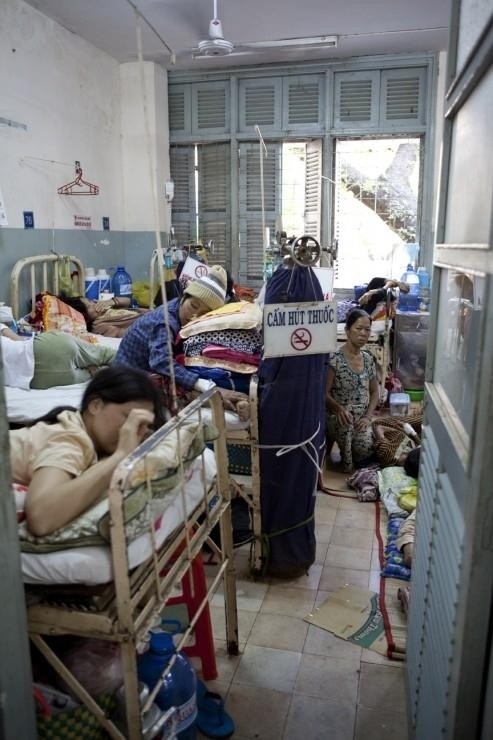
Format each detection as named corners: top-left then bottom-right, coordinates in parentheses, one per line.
top-left (57, 167), bottom-right (99, 195)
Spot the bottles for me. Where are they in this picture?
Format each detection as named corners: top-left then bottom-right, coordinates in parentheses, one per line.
top-left (112, 266), bottom-right (133, 310)
top-left (398, 264), bottom-right (429, 312)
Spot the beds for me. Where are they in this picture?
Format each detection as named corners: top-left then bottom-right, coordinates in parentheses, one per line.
top-left (333, 286), bottom-right (397, 405)
top-left (9, 252), bottom-right (262, 358)
top-left (0, 315), bottom-right (262, 571)
top-left (4, 389), bottom-right (237, 738)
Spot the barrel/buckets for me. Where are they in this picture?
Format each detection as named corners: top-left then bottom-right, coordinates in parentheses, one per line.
top-left (398, 264), bottom-right (420, 310)
top-left (108, 263), bottom-right (134, 300)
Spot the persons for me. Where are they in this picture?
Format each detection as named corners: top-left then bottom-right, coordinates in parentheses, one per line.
top-left (0, 323), bottom-right (118, 391)
top-left (65, 296), bottom-right (153, 338)
top-left (324, 277), bottom-right (423, 567)
top-left (112, 260), bottom-right (249, 412)
top-left (8, 366), bottom-right (165, 537)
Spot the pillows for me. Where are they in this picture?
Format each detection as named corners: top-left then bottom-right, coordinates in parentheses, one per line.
top-left (11, 421), bottom-right (220, 555)
top-left (16, 295), bottom-right (86, 334)
top-left (179, 300), bottom-right (262, 408)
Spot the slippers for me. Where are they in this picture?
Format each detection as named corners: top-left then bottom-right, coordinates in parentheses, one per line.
top-left (194, 675), bottom-right (235, 738)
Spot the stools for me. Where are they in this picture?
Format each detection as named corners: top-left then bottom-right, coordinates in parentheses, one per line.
top-left (138, 528), bottom-right (217, 680)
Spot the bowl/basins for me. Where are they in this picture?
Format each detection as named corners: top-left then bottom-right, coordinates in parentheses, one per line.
top-left (403, 390), bottom-right (426, 402)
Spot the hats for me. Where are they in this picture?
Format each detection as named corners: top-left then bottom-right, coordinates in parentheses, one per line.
top-left (183, 264), bottom-right (227, 310)
